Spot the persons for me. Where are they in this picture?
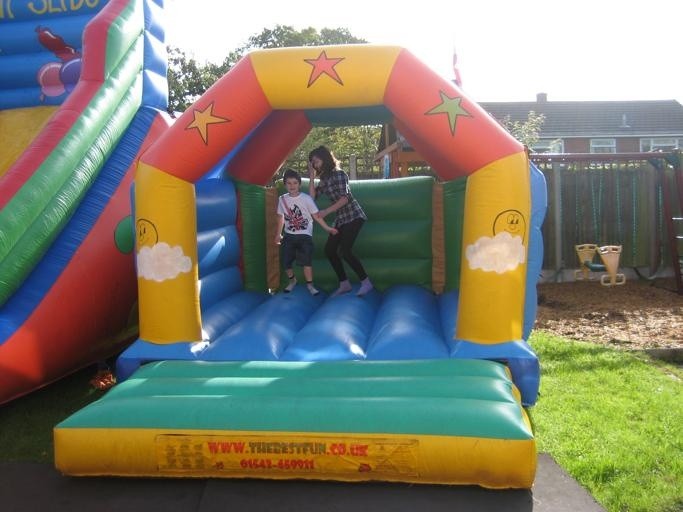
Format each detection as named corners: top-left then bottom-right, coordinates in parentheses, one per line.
top-left (305, 144), bottom-right (373, 297)
top-left (273, 168), bottom-right (340, 296)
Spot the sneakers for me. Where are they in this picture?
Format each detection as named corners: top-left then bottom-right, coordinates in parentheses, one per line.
top-left (88, 370), bottom-right (116, 391)
